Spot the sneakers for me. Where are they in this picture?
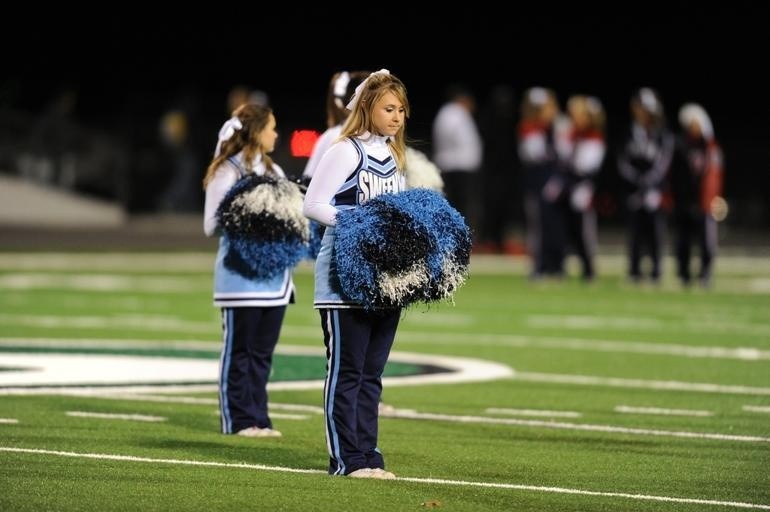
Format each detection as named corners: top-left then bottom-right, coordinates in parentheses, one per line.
top-left (346, 468), bottom-right (396, 480)
top-left (238, 426), bottom-right (282, 437)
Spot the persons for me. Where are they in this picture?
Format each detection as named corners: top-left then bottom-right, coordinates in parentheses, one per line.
top-left (300, 69), bottom-right (410, 480)
top-left (620, 85), bottom-right (723, 288)
top-left (202, 104), bottom-right (290, 437)
top-left (429, 85), bottom-right (482, 227)
top-left (517, 86), bottom-right (606, 288)
